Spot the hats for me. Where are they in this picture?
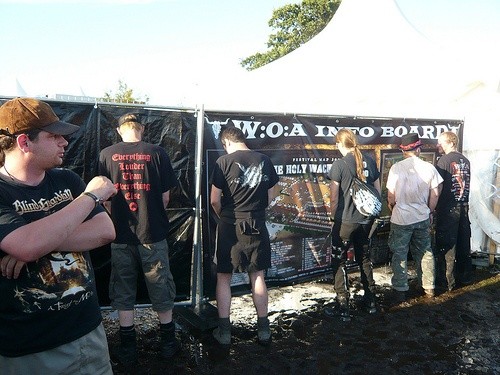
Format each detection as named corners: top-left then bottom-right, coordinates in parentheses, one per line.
top-left (398, 133), bottom-right (423, 151)
top-left (119, 114), bottom-right (141, 126)
top-left (0, 97), bottom-right (80, 136)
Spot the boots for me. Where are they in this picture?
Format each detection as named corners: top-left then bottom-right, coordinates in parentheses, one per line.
top-left (111, 330), bottom-right (137, 365)
top-left (161, 324), bottom-right (180, 357)
top-left (256, 318), bottom-right (272, 343)
top-left (212, 317), bottom-right (231, 344)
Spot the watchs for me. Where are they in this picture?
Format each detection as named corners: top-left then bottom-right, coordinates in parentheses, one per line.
top-left (82, 190), bottom-right (103, 208)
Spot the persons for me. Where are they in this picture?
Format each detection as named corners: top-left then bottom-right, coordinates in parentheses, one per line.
top-left (322, 127), bottom-right (383, 322)
top-left (386, 128), bottom-right (475, 303)
top-left (0, 96), bottom-right (115, 375)
top-left (209, 126), bottom-right (279, 347)
top-left (97, 112), bottom-right (184, 369)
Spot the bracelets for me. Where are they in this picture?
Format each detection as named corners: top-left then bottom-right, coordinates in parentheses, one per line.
top-left (330, 217), bottom-right (336, 223)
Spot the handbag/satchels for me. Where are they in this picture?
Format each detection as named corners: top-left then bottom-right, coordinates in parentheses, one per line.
top-left (352, 177), bottom-right (382, 217)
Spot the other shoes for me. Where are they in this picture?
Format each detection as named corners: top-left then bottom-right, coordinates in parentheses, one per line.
top-left (323, 307), bottom-right (349, 320)
top-left (355, 296), bottom-right (377, 313)
top-left (392, 290), bottom-right (405, 299)
top-left (415, 284), bottom-right (433, 297)
